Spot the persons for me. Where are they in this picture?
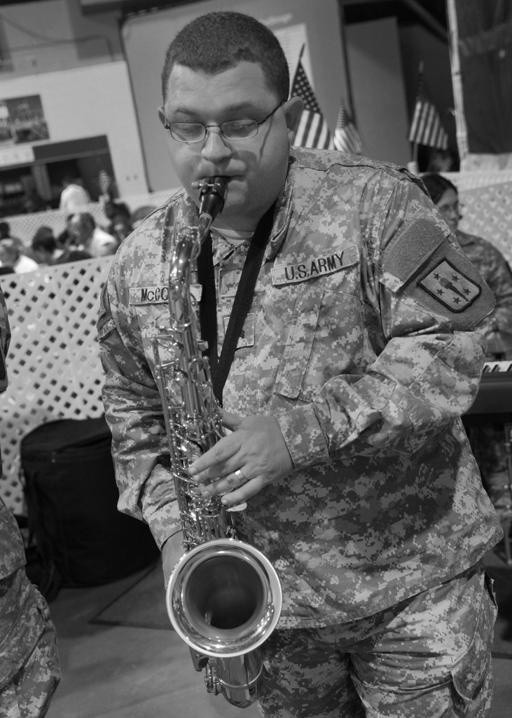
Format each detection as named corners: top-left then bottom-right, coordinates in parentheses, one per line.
top-left (420, 170), bottom-right (510, 364)
top-left (1, 287), bottom-right (62, 718)
top-left (0, 173), bottom-right (132, 276)
top-left (97, 11), bottom-right (496, 717)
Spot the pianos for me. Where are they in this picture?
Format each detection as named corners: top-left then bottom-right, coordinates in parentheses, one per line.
top-left (464, 359), bottom-right (512, 417)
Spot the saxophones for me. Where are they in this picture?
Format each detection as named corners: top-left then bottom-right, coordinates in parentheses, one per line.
top-left (154, 177), bottom-right (283, 709)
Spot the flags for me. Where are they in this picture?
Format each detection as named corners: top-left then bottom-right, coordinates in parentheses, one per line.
top-left (405, 77), bottom-right (451, 151)
top-left (291, 61), bottom-right (335, 149)
top-left (332, 108), bottom-right (363, 154)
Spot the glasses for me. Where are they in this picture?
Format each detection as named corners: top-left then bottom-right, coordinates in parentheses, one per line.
top-left (160, 105), bottom-right (281, 144)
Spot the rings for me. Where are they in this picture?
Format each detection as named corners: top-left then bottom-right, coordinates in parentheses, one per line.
top-left (235, 469), bottom-right (248, 484)
